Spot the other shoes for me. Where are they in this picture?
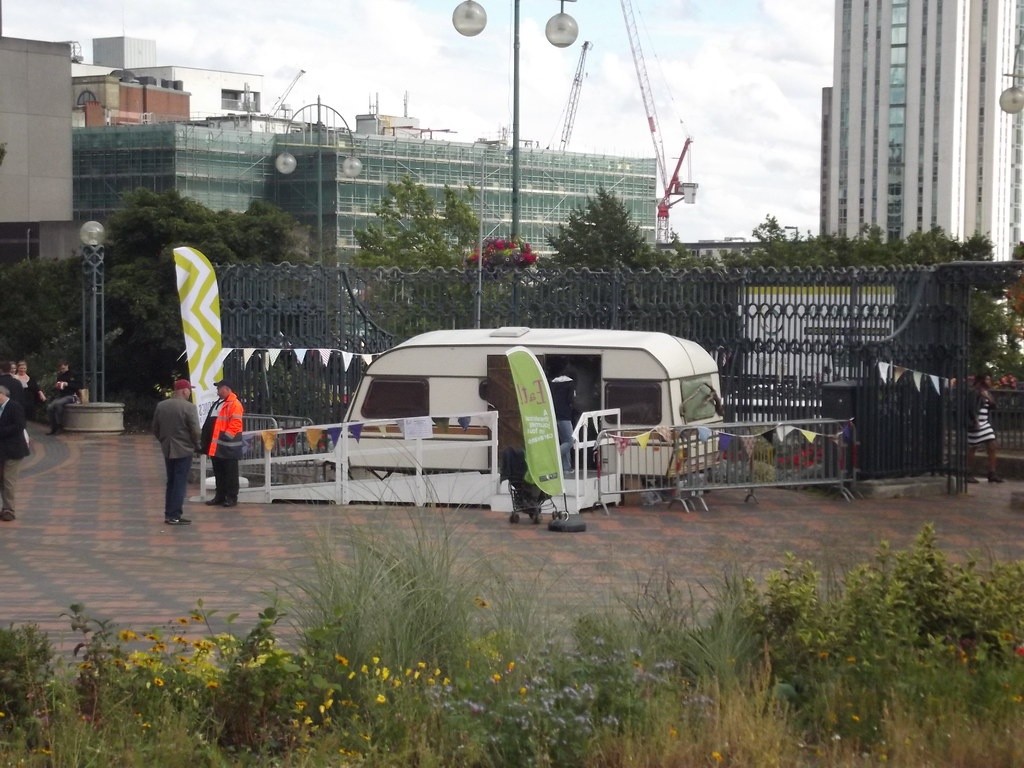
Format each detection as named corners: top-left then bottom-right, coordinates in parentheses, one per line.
top-left (45, 425), bottom-right (55, 435)
top-left (965, 471), bottom-right (979, 483)
top-left (223, 495), bottom-right (238, 506)
top-left (55, 425), bottom-right (63, 435)
top-left (3, 513), bottom-right (14, 521)
top-left (207, 494), bottom-right (224, 505)
top-left (165, 518), bottom-right (192, 524)
top-left (988, 472), bottom-right (1004, 482)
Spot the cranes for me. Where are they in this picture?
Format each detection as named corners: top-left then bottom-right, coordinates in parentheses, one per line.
top-left (558, 41), bottom-right (593, 150)
top-left (620, 0), bottom-right (699, 244)
top-left (270, 70), bottom-right (306, 115)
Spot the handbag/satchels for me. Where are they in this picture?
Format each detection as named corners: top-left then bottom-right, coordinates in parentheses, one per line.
top-left (78, 388), bottom-right (89, 404)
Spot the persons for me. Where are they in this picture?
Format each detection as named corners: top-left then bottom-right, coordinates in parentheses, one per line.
top-left (551, 369), bottom-right (574, 475)
top-left (0, 360), bottom-right (46, 448)
top-left (202, 379), bottom-right (244, 507)
top-left (967, 370), bottom-right (1004, 483)
top-left (0, 385), bottom-right (31, 521)
top-left (46, 361), bottom-right (79, 436)
top-left (152, 379), bottom-right (202, 525)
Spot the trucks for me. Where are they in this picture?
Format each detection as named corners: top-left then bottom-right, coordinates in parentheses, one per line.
top-left (323, 326), bottom-right (724, 504)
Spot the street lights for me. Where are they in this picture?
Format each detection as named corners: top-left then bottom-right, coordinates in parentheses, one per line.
top-left (452, 0), bottom-right (579, 326)
top-left (79, 221), bottom-right (105, 403)
top-left (785, 226), bottom-right (798, 240)
top-left (275, 96), bottom-right (362, 262)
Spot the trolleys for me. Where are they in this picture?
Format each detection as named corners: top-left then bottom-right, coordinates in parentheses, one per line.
top-left (508, 481), bottom-right (562, 524)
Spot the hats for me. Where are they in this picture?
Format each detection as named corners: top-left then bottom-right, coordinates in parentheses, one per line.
top-left (174, 379), bottom-right (195, 390)
top-left (214, 378), bottom-right (233, 388)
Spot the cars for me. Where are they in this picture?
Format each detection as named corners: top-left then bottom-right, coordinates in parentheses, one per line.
top-left (722, 383), bottom-right (821, 422)
